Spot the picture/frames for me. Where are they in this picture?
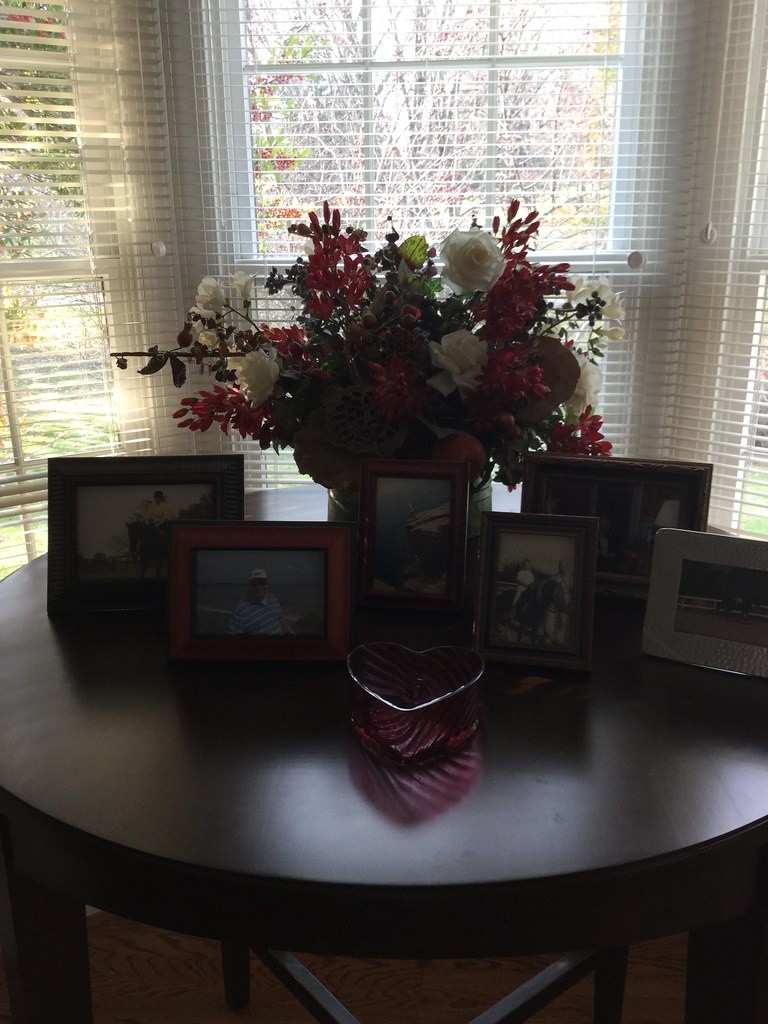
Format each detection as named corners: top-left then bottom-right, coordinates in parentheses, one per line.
top-left (165, 519), bottom-right (356, 667)
top-left (522, 452), bottom-right (713, 600)
top-left (469, 511), bottom-right (600, 672)
top-left (640, 528), bottom-right (768, 679)
top-left (357, 460), bottom-right (471, 607)
top-left (46, 455), bottom-right (244, 614)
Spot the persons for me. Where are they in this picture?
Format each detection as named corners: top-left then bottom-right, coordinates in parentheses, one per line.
top-left (513, 559), bottom-right (574, 644)
top-left (145, 490), bottom-right (169, 532)
top-left (230, 569), bottom-right (293, 636)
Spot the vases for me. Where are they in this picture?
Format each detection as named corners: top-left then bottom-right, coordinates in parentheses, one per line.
top-left (330, 486), bottom-right (491, 611)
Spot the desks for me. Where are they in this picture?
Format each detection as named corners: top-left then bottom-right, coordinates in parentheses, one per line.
top-left (0, 484), bottom-right (768, 1024)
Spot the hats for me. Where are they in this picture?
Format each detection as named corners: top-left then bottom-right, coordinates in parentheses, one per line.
top-left (248, 569), bottom-right (267, 579)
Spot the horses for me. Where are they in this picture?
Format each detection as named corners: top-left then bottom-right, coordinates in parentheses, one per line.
top-left (124, 522), bottom-right (168, 576)
top-left (715, 590), bottom-right (752, 621)
top-left (502, 583), bottom-right (568, 644)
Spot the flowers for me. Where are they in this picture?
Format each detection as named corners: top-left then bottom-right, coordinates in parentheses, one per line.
top-left (111, 196), bottom-right (614, 497)
